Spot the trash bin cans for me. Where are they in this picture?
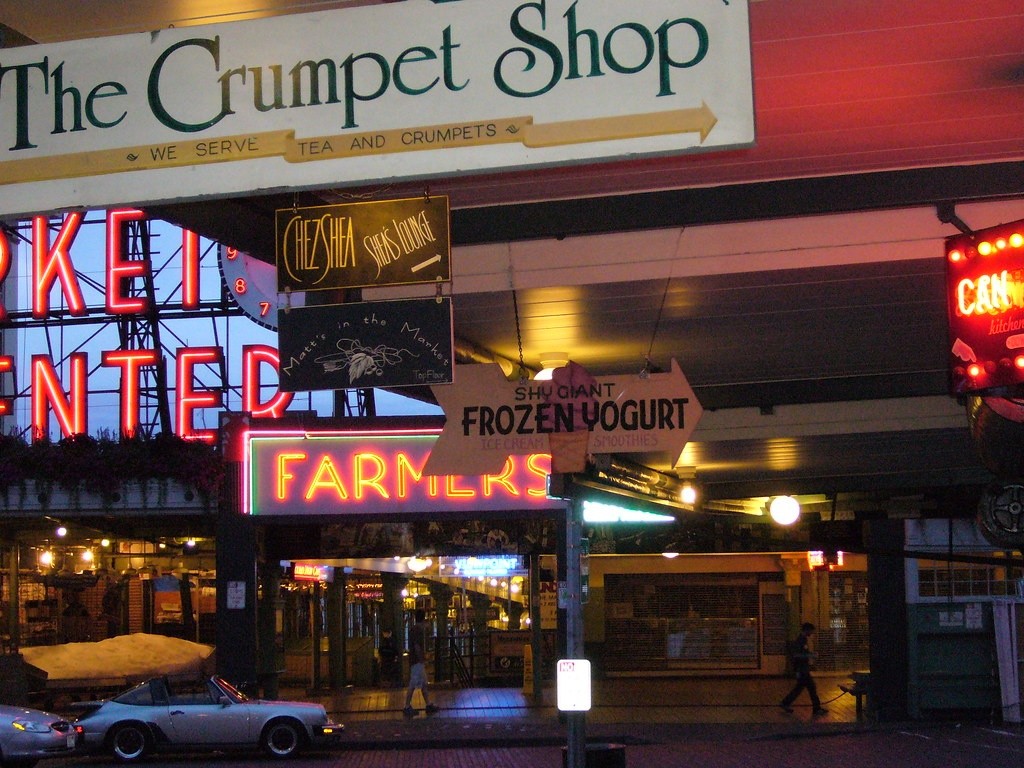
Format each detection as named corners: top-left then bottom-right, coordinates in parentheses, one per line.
top-left (248, 673), bottom-right (271, 699)
top-left (561, 743), bottom-right (626, 768)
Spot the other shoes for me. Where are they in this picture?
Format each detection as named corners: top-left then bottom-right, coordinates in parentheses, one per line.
top-left (403, 705), bottom-right (419, 714)
top-left (779, 701), bottom-right (794, 713)
top-left (426, 705), bottom-right (439, 713)
top-left (813, 708), bottom-right (829, 717)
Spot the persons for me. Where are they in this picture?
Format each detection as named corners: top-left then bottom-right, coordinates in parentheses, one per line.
top-left (379, 628), bottom-right (399, 689)
top-left (402, 609), bottom-right (439, 716)
top-left (780, 622), bottom-right (828, 714)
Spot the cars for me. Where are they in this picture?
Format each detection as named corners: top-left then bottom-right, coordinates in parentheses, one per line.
top-left (0, 704), bottom-right (77, 768)
top-left (71, 671), bottom-right (345, 764)
